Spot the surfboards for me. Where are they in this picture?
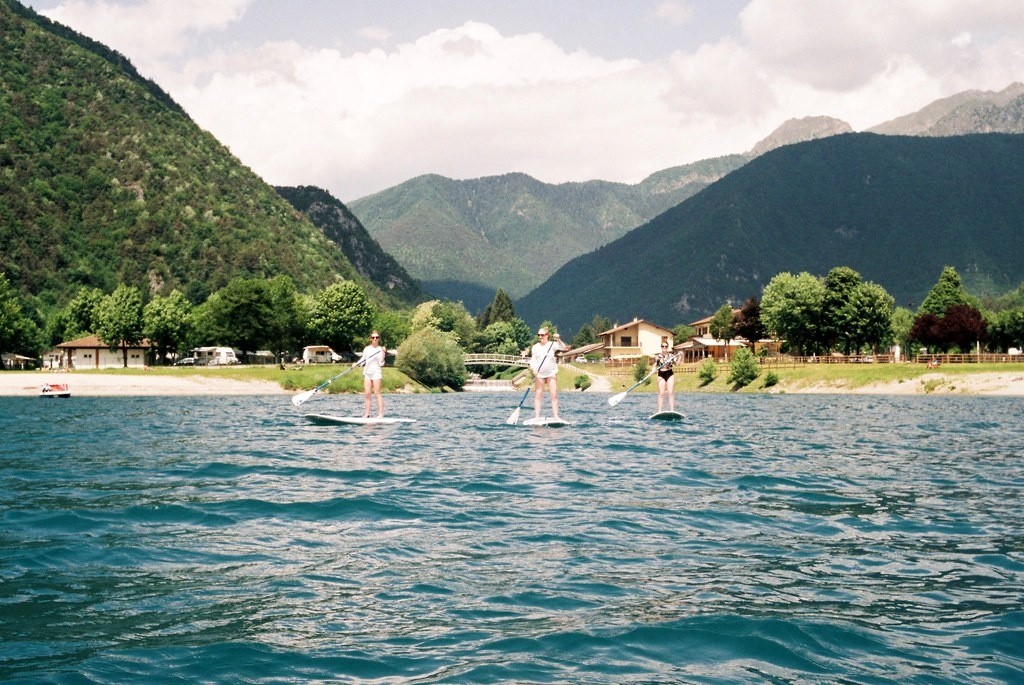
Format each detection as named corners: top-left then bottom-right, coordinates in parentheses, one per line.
top-left (304, 412), bottom-right (419, 426)
top-left (648, 410), bottom-right (686, 422)
top-left (521, 415), bottom-right (568, 427)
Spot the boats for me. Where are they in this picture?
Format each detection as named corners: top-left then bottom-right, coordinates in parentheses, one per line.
top-left (39, 384), bottom-right (71, 398)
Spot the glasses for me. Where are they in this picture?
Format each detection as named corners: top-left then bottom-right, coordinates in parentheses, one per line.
top-left (537, 333), bottom-right (547, 337)
top-left (371, 336), bottom-right (379, 339)
top-left (661, 346), bottom-right (668, 348)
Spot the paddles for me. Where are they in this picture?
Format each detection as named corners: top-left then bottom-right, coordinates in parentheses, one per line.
top-left (607, 352), bottom-right (680, 408)
top-left (505, 337), bottom-right (555, 427)
top-left (291, 348), bottom-right (381, 407)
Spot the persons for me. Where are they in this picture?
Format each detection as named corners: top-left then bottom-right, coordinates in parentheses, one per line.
top-left (352, 331), bottom-right (386, 418)
top-left (652, 341), bottom-right (684, 412)
top-left (531, 326), bottom-right (566, 419)
